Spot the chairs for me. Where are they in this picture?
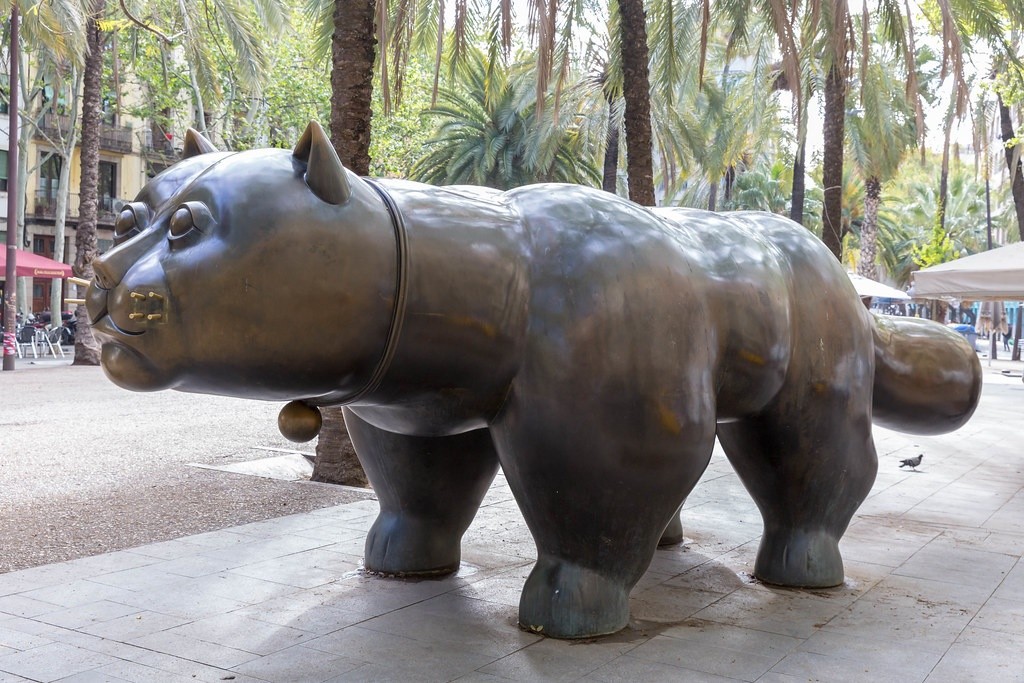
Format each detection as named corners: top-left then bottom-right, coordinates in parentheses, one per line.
top-left (16, 326), bottom-right (66, 358)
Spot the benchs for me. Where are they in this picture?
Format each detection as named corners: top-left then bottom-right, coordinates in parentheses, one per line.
top-left (1017, 339), bottom-right (1024, 360)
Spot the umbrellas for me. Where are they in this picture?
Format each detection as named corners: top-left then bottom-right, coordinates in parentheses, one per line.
top-left (914, 238), bottom-right (1024, 304)
top-left (846, 269), bottom-right (911, 301)
top-left (0, 243), bottom-right (72, 279)
top-left (975, 299), bottom-right (1009, 359)
